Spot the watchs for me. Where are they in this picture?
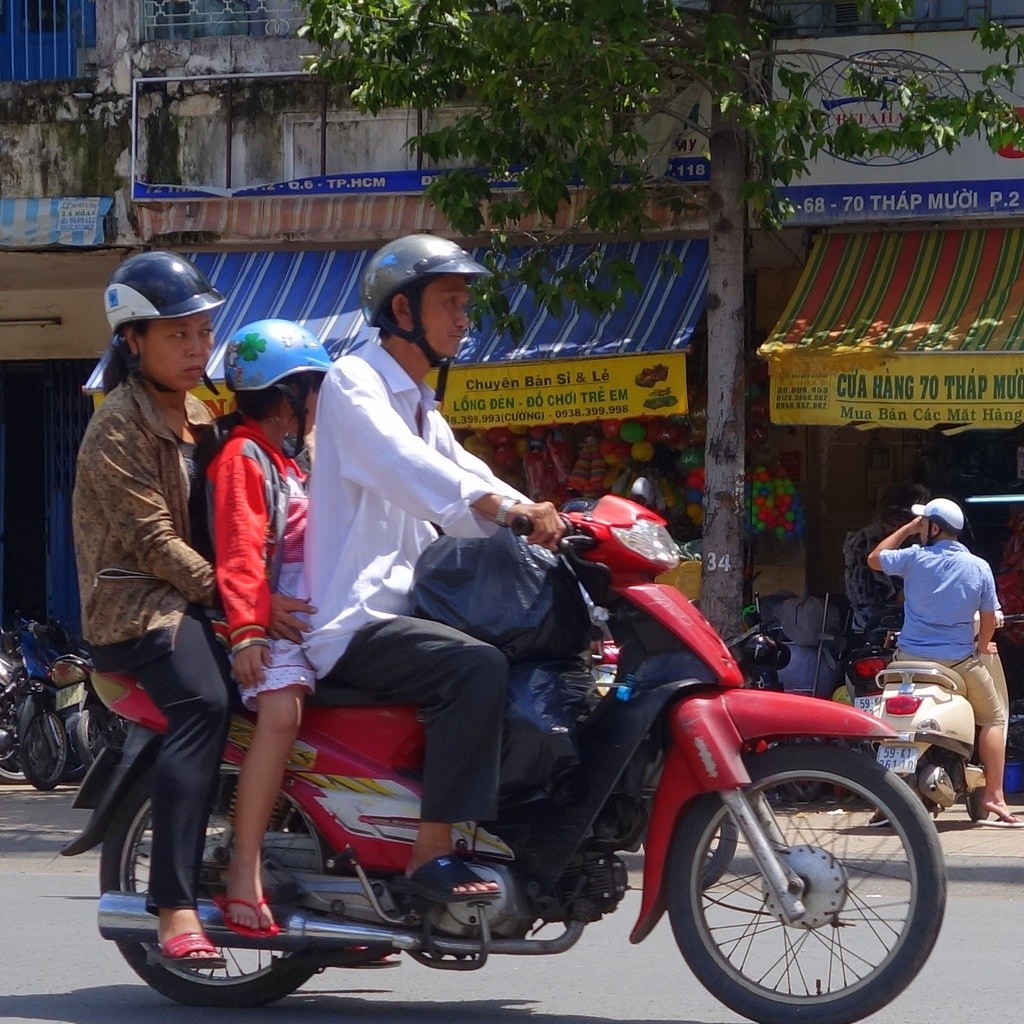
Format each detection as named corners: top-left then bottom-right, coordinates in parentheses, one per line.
top-left (494, 496), bottom-right (522, 528)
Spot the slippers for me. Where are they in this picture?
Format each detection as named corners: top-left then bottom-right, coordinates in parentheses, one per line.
top-left (388, 854), bottom-right (502, 901)
top-left (211, 890), bottom-right (281, 937)
top-left (148, 933), bottom-right (228, 968)
top-left (868, 813), bottom-right (890, 826)
top-left (976, 813), bottom-right (1024, 827)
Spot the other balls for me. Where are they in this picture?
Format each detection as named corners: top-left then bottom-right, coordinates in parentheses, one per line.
top-left (464, 423), bottom-right (545, 464)
top-left (599, 419), bottom-right (703, 472)
top-left (743, 459), bottom-right (806, 543)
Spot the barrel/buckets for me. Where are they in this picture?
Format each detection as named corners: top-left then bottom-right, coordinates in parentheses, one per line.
top-left (1004, 759), bottom-right (1024, 793)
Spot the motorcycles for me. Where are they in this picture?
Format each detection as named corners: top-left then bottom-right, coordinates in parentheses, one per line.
top-left (58, 474), bottom-right (946, 1024)
top-left (863, 609), bottom-right (1024, 818)
top-left (818, 615), bottom-right (901, 758)
top-left (711, 589), bottom-right (825, 807)
top-left (0, 610), bottom-right (122, 790)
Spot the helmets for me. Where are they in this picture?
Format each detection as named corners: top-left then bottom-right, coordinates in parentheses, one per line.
top-left (362, 234), bottom-right (494, 326)
top-left (223, 319), bottom-right (332, 391)
top-left (105, 251), bottom-right (227, 336)
top-left (912, 498), bottom-right (964, 535)
top-left (742, 632), bottom-right (792, 670)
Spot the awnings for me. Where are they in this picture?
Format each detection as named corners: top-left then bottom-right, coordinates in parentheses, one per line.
top-left (81, 231), bottom-right (713, 430)
top-left (756, 220), bottom-right (1024, 428)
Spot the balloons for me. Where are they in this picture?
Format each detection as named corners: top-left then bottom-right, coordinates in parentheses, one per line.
top-left (463, 379), bottom-right (767, 524)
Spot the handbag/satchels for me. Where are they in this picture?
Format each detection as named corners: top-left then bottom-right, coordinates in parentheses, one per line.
top-left (407, 521), bottom-right (590, 668)
top-left (494, 655), bottom-right (601, 810)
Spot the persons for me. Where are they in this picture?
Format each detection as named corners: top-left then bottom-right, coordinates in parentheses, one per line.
top-left (69, 234), bottom-right (567, 971)
top-left (868, 497), bottom-right (1024, 828)
